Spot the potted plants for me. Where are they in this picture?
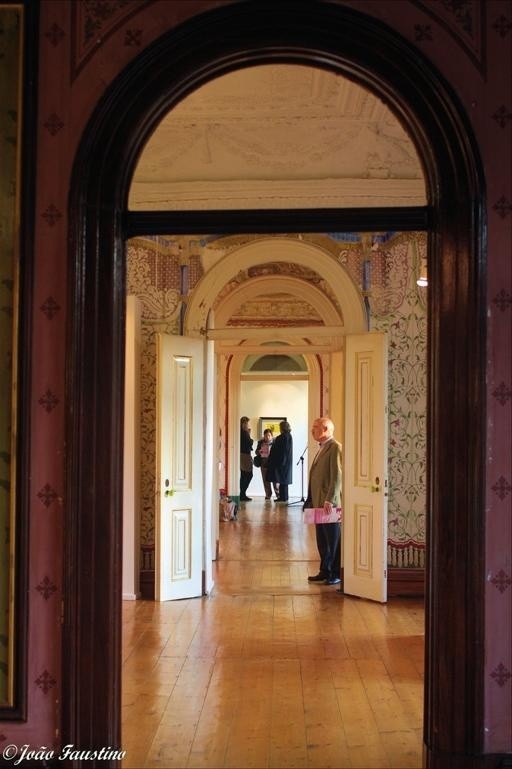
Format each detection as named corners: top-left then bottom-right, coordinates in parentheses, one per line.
top-left (258, 417), bottom-right (287, 439)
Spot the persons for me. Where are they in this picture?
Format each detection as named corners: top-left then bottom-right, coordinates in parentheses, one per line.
top-left (304, 417), bottom-right (343, 586)
top-left (253, 429), bottom-right (284, 501)
top-left (240, 416), bottom-right (255, 501)
top-left (264, 422), bottom-right (293, 503)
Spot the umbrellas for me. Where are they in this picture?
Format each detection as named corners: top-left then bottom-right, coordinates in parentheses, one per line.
top-left (274, 497), bottom-right (289, 502)
top-left (240, 496), bottom-right (252, 501)
top-left (307, 572), bottom-right (341, 585)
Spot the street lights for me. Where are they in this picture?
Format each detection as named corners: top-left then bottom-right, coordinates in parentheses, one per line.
top-left (254, 456), bottom-right (260, 467)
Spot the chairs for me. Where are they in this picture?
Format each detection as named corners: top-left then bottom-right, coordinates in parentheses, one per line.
top-left (265, 493), bottom-right (272, 499)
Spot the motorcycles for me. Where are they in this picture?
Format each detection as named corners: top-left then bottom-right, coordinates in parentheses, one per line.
top-left (285, 447), bottom-right (308, 506)
